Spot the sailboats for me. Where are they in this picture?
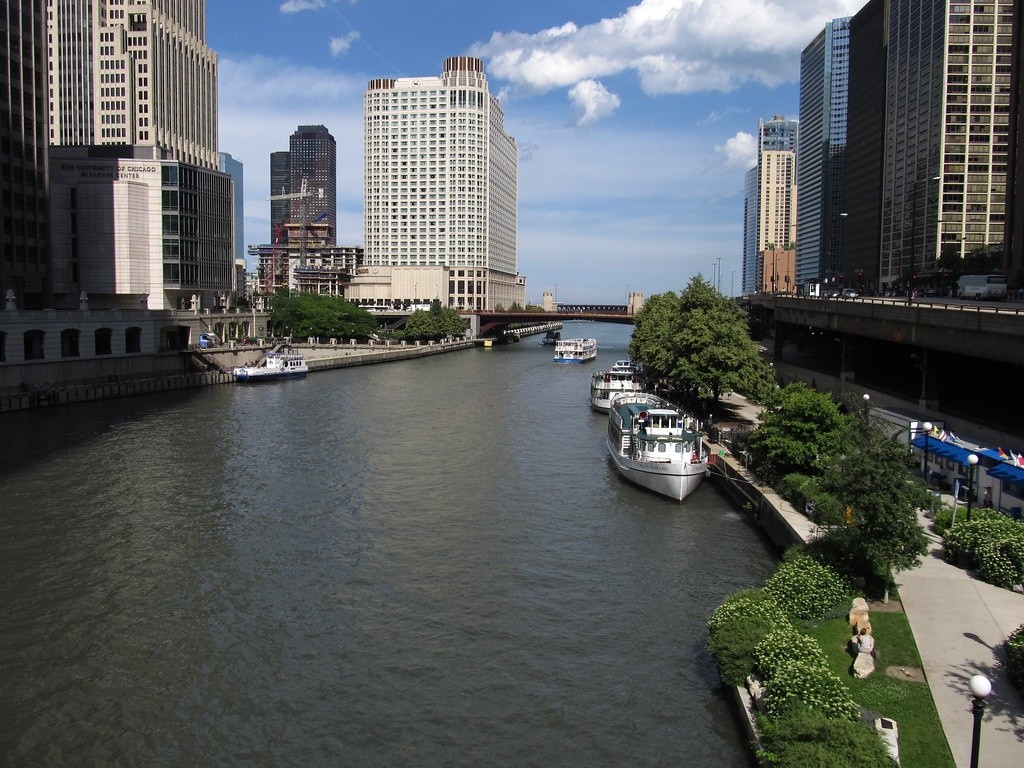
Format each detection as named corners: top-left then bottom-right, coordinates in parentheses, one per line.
top-left (234, 340), bottom-right (310, 380)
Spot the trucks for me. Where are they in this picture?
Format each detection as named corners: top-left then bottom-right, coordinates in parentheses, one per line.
top-left (406, 304), bottom-right (431, 312)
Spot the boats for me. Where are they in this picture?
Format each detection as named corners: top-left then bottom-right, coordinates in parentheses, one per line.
top-left (605, 389), bottom-right (711, 502)
top-left (472, 320), bottom-right (520, 348)
top-left (553, 336), bottom-right (598, 366)
top-left (542, 329), bottom-right (561, 343)
top-left (589, 353), bottom-right (639, 413)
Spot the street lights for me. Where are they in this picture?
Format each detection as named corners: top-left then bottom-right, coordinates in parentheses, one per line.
top-left (732, 270), bottom-right (737, 299)
top-left (415, 282), bottom-right (420, 304)
top-left (555, 282), bottom-right (559, 303)
top-left (787, 245), bottom-right (794, 297)
top-left (766, 262), bottom-right (773, 293)
top-left (625, 283), bottom-right (627, 305)
top-left (776, 251), bottom-right (785, 295)
top-left (776, 257), bottom-right (782, 296)
top-left (628, 281), bottom-right (631, 304)
top-left (716, 256), bottom-right (721, 293)
top-left (436, 284), bottom-right (439, 300)
top-left (712, 262), bottom-right (716, 290)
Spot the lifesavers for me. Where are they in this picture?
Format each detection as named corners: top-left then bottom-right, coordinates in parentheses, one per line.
top-left (636, 451), bottom-right (641, 460)
